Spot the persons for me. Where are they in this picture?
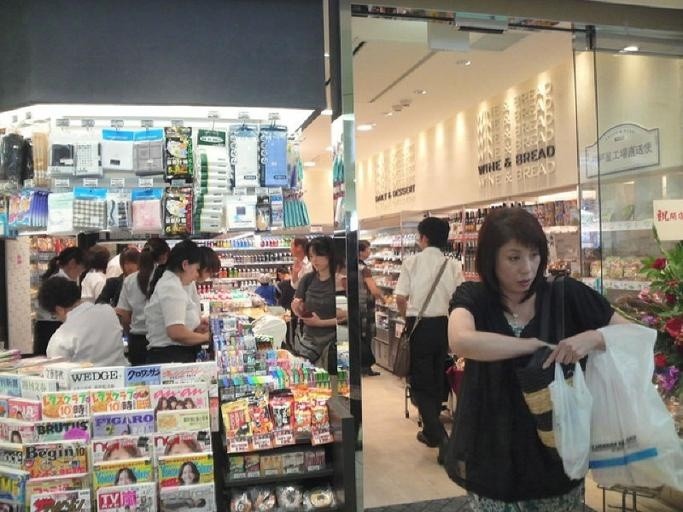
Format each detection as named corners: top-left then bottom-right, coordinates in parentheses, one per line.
top-left (34, 231), bottom-right (224, 371)
top-left (444, 205), bottom-right (637, 512)
top-left (394, 216), bottom-right (466, 466)
top-left (252, 234), bottom-right (389, 379)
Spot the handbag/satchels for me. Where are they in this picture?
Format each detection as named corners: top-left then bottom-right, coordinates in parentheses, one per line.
top-left (516, 340), bottom-right (588, 449)
top-left (294, 327), bottom-right (324, 363)
top-left (387, 335), bottom-right (411, 377)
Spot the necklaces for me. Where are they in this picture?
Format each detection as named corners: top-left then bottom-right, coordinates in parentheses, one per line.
top-left (511, 304), bottom-right (519, 318)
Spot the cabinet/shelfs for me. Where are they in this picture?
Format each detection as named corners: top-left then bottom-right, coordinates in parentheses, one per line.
top-left (358, 182), bottom-right (596, 328)
top-left (195, 239), bottom-right (294, 326)
top-left (28, 233), bottom-right (76, 320)
top-left (211, 395), bottom-right (358, 510)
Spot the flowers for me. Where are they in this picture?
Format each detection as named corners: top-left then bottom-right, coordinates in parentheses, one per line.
top-left (613, 221), bottom-right (681, 400)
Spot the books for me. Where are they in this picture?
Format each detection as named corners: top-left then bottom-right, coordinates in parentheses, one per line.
top-left (0, 348), bottom-right (222, 511)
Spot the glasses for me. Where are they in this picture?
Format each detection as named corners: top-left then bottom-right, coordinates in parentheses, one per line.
top-left (51, 313), bottom-right (56, 320)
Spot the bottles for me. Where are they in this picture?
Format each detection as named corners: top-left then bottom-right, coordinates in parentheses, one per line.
top-left (221, 252), bottom-right (292, 264)
top-left (197, 280), bottom-right (260, 299)
top-left (446, 201), bottom-right (526, 234)
top-left (214, 266), bottom-right (291, 279)
top-left (202, 236), bottom-right (292, 248)
top-left (442, 238), bottom-right (479, 276)
top-left (267, 366), bottom-right (308, 390)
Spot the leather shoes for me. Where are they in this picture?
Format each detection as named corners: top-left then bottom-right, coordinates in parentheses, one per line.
top-left (417, 431), bottom-right (437, 447)
top-left (438, 436), bottom-right (450, 464)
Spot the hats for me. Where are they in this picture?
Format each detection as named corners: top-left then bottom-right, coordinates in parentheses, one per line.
top-left (258, 274), bottom-right (270, 283)
top-left (276, 267), bottom-right (288, 281)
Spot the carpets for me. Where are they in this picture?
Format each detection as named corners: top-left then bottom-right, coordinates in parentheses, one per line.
top-left (369, 493), bottom-right (596, 512)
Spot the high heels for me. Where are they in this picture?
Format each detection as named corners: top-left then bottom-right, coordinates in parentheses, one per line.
top-left (361, 366), bottom-right (380, 378)
